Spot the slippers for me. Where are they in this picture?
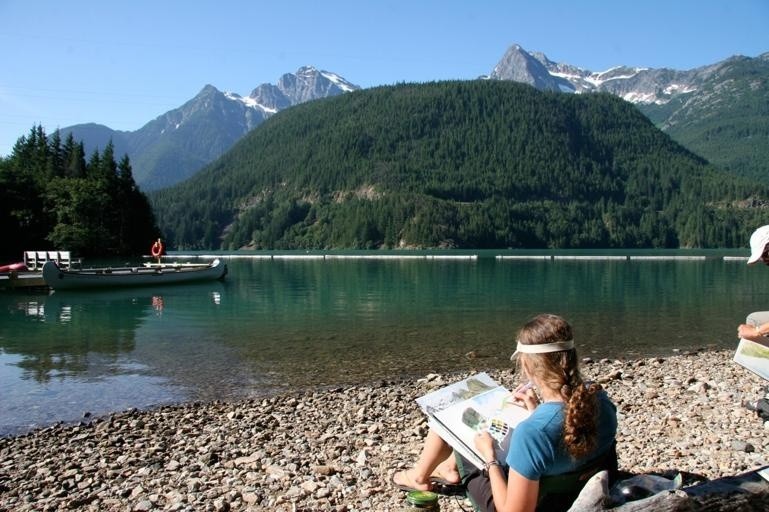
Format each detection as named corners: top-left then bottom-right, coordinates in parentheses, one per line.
top-left (384, 467), bottom-right (459, 494)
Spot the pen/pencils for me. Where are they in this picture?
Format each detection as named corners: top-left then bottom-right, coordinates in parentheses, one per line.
top-left (508, 383), bottom-right (533, 401)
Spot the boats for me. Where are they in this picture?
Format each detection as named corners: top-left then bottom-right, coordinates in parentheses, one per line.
top-left (0, 236), bottom-right (227, 291)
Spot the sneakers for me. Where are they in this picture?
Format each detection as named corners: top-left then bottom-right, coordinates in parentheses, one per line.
top-left (747, 400), bottom-right (759, 410)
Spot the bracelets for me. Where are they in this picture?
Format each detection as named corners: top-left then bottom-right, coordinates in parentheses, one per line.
top-left (483, 459), bottom-right (501, 473)
top-left (756, 326), bottom-right (764, 337)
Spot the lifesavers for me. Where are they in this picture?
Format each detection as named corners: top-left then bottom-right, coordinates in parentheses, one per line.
top-left (152, 242), bottom-right (162, 257)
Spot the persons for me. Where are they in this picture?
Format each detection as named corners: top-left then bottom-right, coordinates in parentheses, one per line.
top-left (737, 225), bottom-right (768, 411)
top-left (390, 313), bottom-right (618, 511)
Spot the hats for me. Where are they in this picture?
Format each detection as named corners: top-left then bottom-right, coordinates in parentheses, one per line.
top-left (747, 224), bottom-right (769, 265)
top-left (510, 335), bottom-right (575, 361)
